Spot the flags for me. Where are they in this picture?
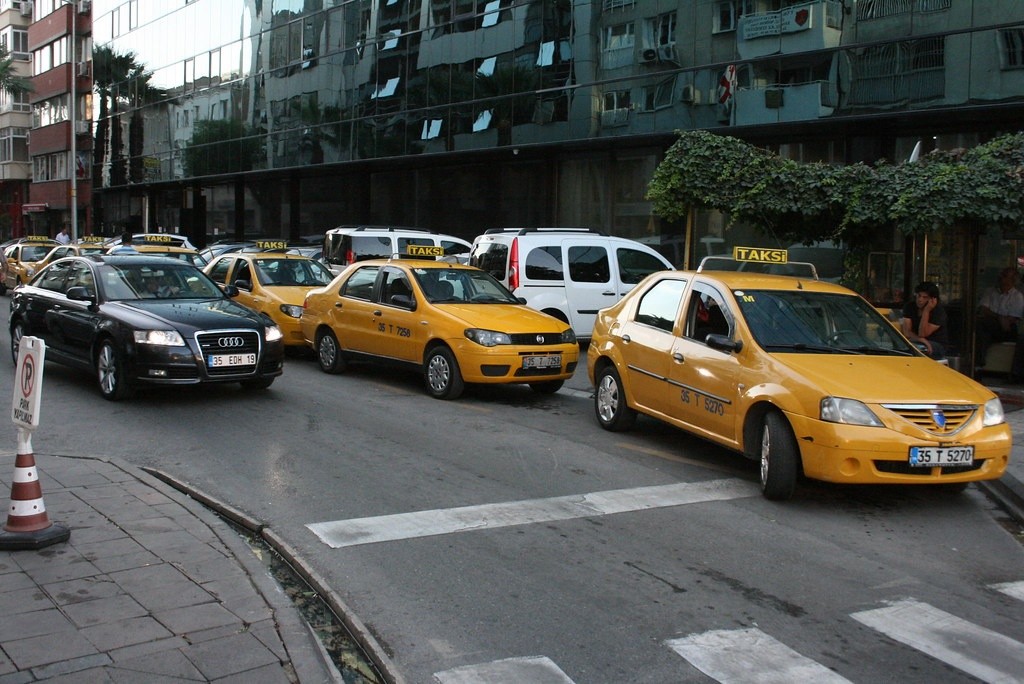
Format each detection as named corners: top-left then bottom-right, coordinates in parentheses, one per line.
top-left (718, 65), bottom-right (739, 112)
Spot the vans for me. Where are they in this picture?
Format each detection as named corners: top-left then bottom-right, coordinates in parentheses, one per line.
top-left (320, 225), bottom-right (472, 278)
top-left (470, 226), bottom-right (678, 342)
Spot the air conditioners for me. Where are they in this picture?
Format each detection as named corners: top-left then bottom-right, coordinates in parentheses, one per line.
top-left (77, 0), bottom-right (88, 14)
top-left (77, 61), bottom-right (87, 76)
top-left (76, 121), bottom-right (88, 134)
top-left (677, 84), bottom-right (693, 102)
top-left (639, 48), bottom-right (658, 63)
top-left (20, 2), bottom-right (32, 17)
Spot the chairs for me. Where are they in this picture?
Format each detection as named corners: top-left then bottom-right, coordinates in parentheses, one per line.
top-left (697, 304), bottom-right (728, 347)
top-left (276, 268), bottom-right (299, 283)
top-left (386, 278), bottom-right (412, 301)
top-left (432, 280), bottom-right (456, 298)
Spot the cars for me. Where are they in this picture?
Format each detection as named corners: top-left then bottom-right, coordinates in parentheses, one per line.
top-left (0, 236), bottom-right (66, 296)
top-left (34, 244), bottom-right (208, 280)
top-left (187, 251), bottom-right (335, 345)
top-left (864, 308), bottom-right (903, 341)
top-left (7, 255), bottom-right (284, 403)
top-left (78, 234), bottom-right (112, 243)
top-left (193, 238), bottom-right (324, 267)
top-left (303, 248), bottom-right (582, 400)
top-left (586, 248), bottom-right (1012, 499)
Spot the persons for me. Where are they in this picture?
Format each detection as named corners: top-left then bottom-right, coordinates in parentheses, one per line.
top-left (902, 282), bottom-right (948, 356)
top-left (112, 232), bottom-right (142, 286)
top-left (56, 228), bottom-right (69, 244)
top-left (975, 268), bottom-right (1024, 366)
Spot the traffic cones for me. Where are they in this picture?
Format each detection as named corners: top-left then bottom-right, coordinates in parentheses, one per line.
top-left (0, 427), bottom-right (72, 554)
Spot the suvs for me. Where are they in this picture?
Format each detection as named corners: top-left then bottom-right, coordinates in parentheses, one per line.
top-left (103, 232), bottom-right (199, 250)
